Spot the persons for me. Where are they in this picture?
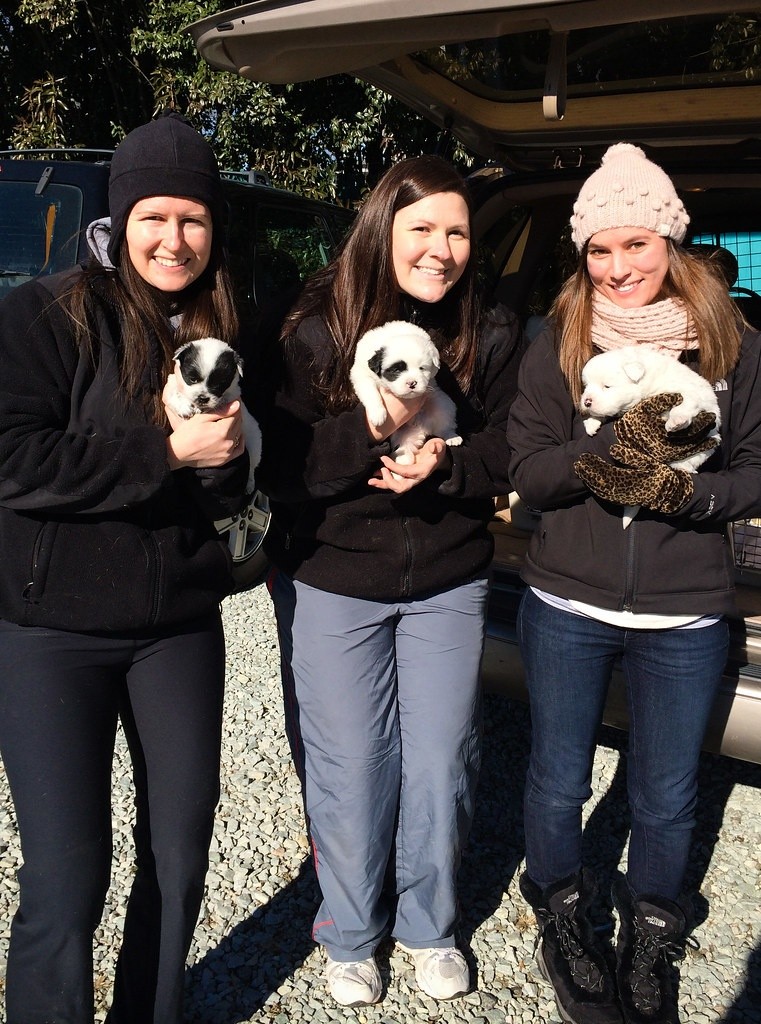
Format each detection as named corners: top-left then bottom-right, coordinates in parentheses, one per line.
top-left (1, 115), bottom-right (314, 1024)
top-left (506, 142), bottom-right (761, 1024)
top-left (264, 154), bottom-right (528, 1007)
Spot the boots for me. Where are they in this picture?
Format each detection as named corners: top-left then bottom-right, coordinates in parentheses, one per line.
top-left (612, 870), bottom-right (708, 1024)
top-left (519, 868), bottom-right (623, 1024)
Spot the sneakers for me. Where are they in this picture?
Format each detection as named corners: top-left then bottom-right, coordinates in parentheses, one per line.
top-left (391, 937), bottom-right (469, 999)
top-left (325, 956), bottom-right (382, 1007)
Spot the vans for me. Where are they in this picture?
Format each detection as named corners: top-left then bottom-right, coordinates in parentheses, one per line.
top-left (0, 148), bottom-right (370, 300)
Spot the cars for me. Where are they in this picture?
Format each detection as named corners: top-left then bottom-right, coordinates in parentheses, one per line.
top-left (173, 0), bottom-right (760, 764)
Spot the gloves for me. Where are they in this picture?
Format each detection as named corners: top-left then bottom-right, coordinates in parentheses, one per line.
top-left (573, 444), bottom-right (693, 515)
top-left (614, 393), bottom-right (719, 464)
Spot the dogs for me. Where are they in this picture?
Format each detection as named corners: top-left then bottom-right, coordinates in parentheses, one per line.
top-left (162, 335), bottom-right (262, 497)
top-left (349, 319), bottom-right (462, 482)
top-left (579, 343), bottom-right (720, 529)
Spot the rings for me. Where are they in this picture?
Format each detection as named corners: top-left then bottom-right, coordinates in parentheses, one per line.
top-left (235, 437), bottom-right (239, 447)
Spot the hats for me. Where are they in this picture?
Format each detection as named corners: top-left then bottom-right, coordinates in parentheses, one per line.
top-left (569, 142), bottom-right (690, 256)
top-left (107, 107), bottom-right (228, 266)
